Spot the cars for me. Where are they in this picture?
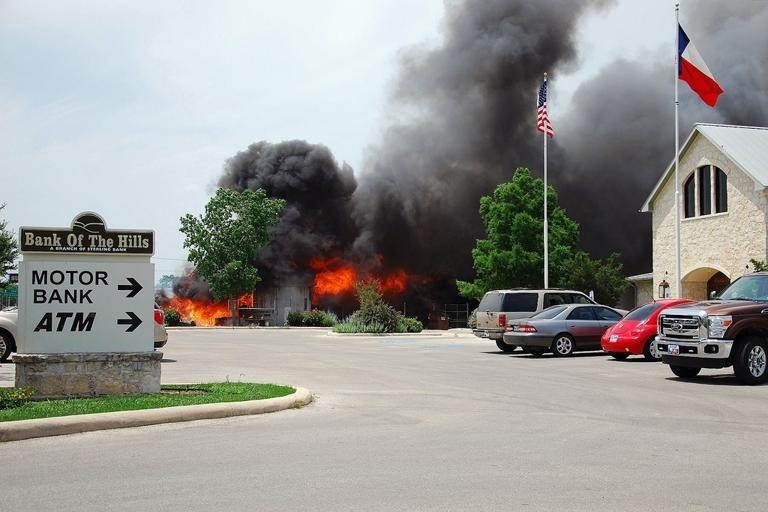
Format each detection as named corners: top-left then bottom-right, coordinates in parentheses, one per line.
top-left (473, 272), bottom-right (768, 385)
top-left (0, 297), bottom-right (170, 362)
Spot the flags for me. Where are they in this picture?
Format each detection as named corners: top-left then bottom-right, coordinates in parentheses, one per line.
top-left (678, 22), bottom-right (725, 108)
top-left (536, 79), bottom-right (555, 139)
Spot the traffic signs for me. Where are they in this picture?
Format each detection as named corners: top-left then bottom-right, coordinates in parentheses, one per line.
top-left (19, 252), bottom-right (156, 352)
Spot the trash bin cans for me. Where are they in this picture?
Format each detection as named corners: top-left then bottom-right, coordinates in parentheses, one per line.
top-left (427, 312), bottom-right (449, 330)
